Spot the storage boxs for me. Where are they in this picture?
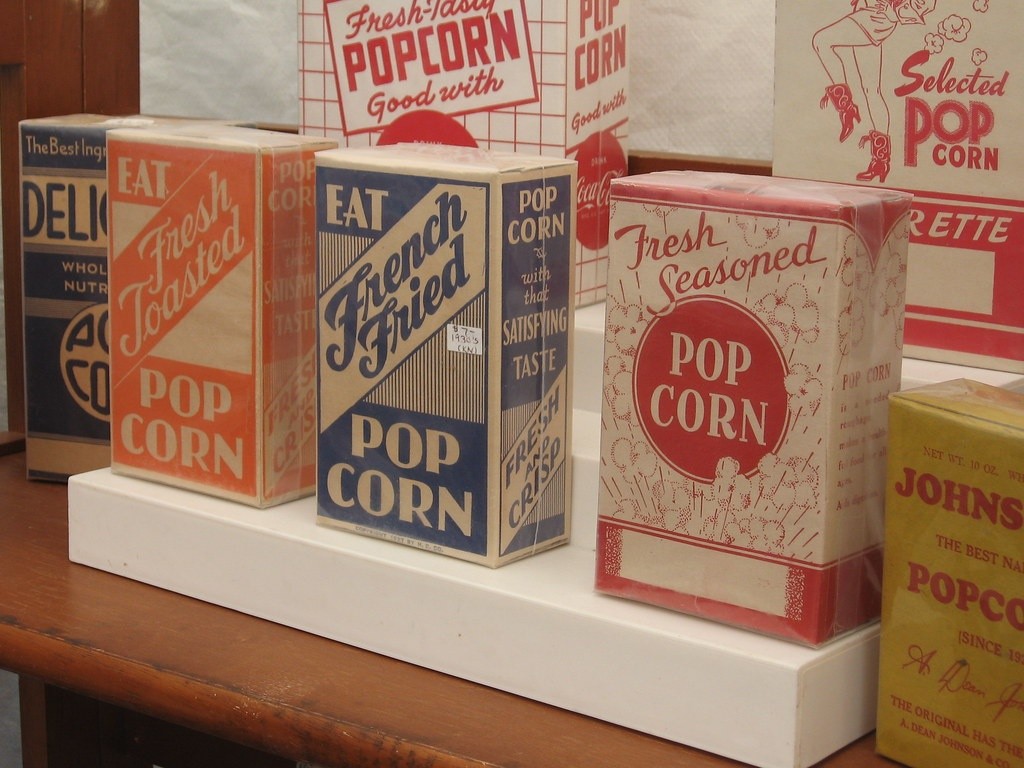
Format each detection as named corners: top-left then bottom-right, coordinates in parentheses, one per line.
top-left (18, 0), bottom-right (1023, 768)
